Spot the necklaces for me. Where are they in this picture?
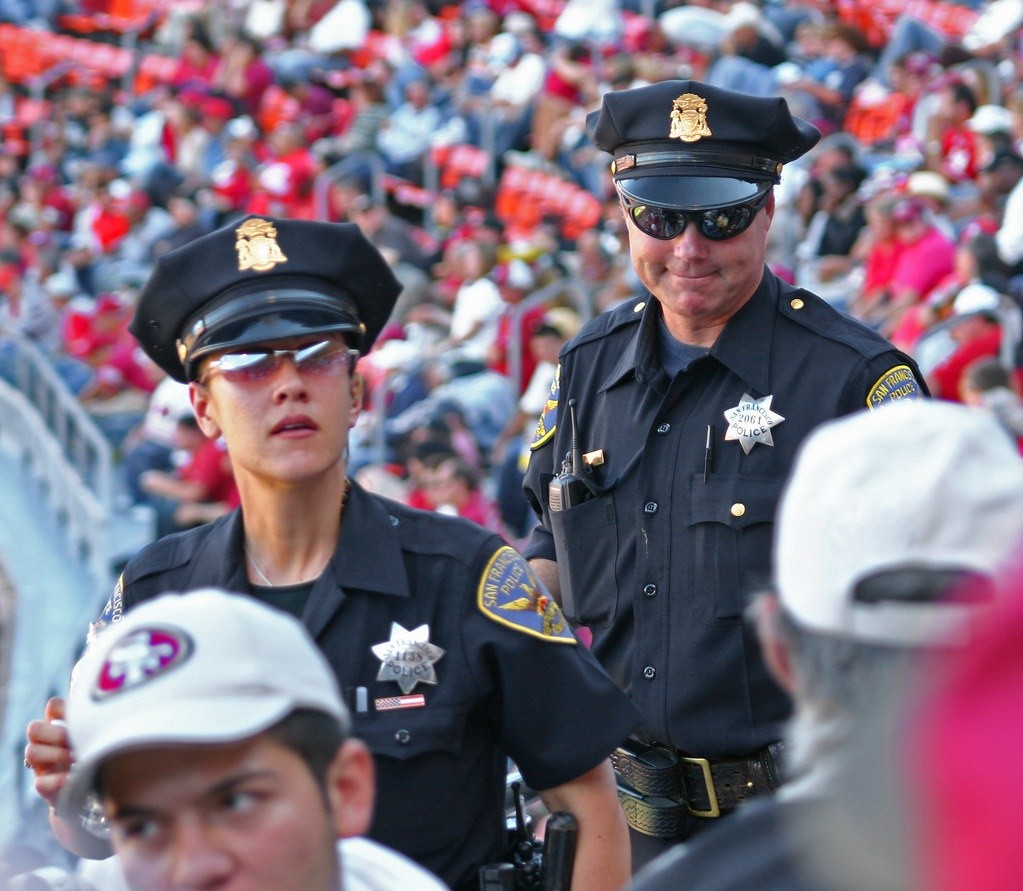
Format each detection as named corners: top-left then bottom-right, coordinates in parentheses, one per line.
top-left (241, 544), bottom-right (272, 587)
top-left (339, 477), bottom-right (352, 521)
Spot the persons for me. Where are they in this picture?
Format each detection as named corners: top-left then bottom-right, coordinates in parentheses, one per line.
top-left (0, 586), bottom-right (450, 891)
top-left (1, 0), bottom-right (1023, 538)
top-left (512, 77), bottom-right (935, 883)
top-left (605, 396), bottom-right (1023, 891)
top-left (22, 213), bottom-right (640, 891)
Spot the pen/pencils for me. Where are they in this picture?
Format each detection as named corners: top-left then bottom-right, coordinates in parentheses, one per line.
top-left (703, 424), bottom-right (715, 484)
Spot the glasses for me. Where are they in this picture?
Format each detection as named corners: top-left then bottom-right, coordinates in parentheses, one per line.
top-left (196, 338), bottom-right (362, 384)
top-left (618, 193), bottom-right (771, 241)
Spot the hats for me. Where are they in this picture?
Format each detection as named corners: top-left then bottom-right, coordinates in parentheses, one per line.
top-left (586, 80), bottom-right (823, 209)
top-left (54, 586), bottom-right (351, 816)
top-left (770, 395), bottom-right (1022, 650)
top-left (125, 212), bottom-right (404, 385)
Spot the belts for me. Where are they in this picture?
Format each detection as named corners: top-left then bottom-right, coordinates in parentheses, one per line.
top-left (611, 740), bottom-right (794, 839)
